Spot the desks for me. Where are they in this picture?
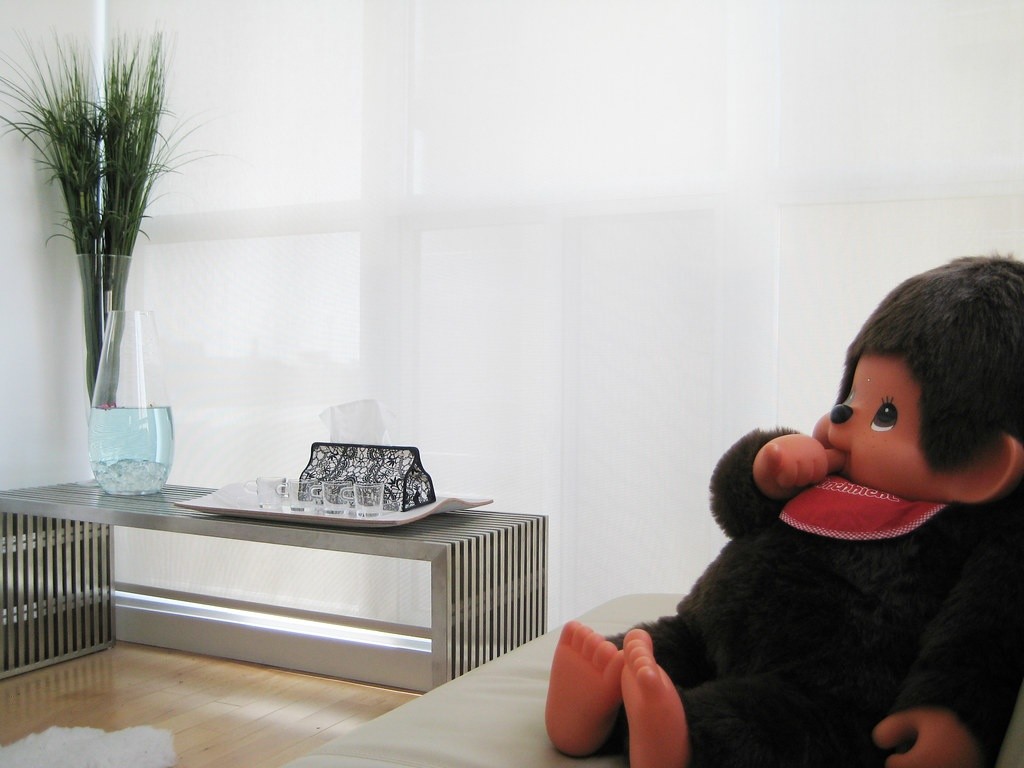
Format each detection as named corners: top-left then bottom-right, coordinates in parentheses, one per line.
top-left (0, 481), bottom-right (548, 695)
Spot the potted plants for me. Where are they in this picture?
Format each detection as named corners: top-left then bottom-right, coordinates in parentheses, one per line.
top-left (7, 26), bottom-right (235, 402)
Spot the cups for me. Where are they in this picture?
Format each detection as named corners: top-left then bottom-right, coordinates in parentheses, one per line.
top-left (274, 480), bottom-right (317, 513)
top-left (309, 481), bottom-right (352, 515)
top-left (340, 483), bottom-right (384, 518)
top-left (246, 477), bottom-right (286, 510)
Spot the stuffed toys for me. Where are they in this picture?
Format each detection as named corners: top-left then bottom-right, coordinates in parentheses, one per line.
top-left (545, 252), bottom-right (1024, 768)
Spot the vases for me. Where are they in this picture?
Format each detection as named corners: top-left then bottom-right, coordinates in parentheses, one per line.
top-left (88, 309), bottom-right (172, 496)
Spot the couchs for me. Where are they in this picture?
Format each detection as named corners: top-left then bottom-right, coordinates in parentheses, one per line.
top-left (285, 588), bottom-right (1024, 768)
